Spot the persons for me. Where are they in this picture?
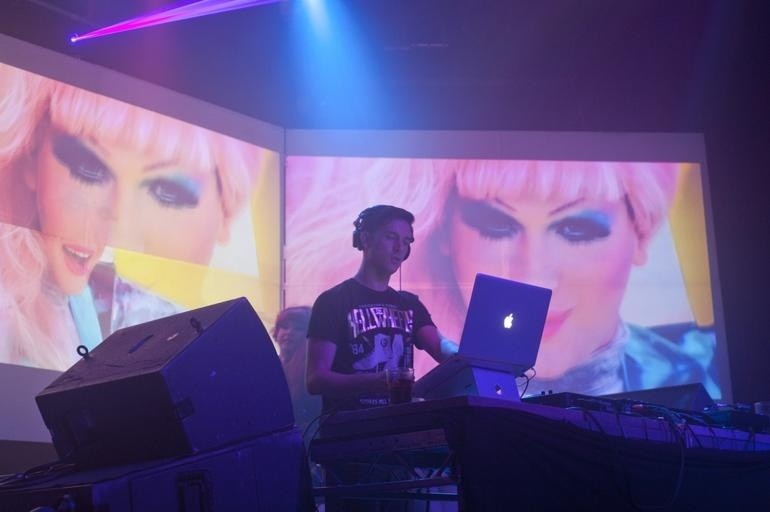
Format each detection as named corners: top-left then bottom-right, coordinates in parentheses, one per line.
top-left (282, 156), bottom-right (724, 404)
top-left (306, 205), bottom-right (460, 512)
top-left (1, 64), bottom-right (254, 271)
top-left (1, 222), bottom-right (107, 373)
top-left (269, 303), bottom-right (315, 425)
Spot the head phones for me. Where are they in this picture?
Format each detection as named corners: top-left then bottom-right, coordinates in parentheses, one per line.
top-left (352, 204), bottom-right (415, 262)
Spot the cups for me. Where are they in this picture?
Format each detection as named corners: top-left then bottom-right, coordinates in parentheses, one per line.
top-left (386, 368), bottom-right (414, 407)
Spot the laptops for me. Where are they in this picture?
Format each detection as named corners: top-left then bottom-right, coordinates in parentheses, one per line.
top-left (412, 273), bottom-right (553, 399)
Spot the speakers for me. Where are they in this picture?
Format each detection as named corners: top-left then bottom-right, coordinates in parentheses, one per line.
top-left (0, 426), bottom-right (318, 512)
top-left (35, 296), bottom-right (297, 465)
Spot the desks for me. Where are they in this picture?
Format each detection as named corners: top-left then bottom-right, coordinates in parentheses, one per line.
top-left (307, 396), bottom-right (770, 512)
